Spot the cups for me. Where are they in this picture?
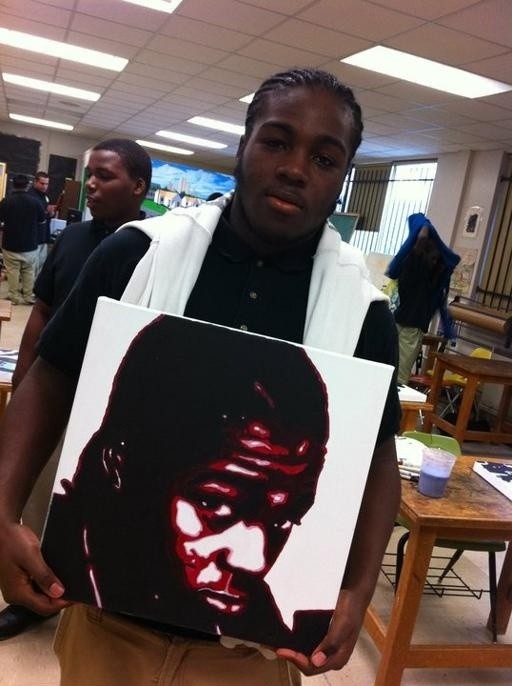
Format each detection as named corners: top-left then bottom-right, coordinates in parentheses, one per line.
top-left (417, 447), bottom-right (458, 498)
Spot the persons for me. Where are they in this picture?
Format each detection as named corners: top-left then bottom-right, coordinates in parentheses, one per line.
top-left (13, 138), bottom-right (153, 395)
top-left (20, 172), bottom-right (58, 295)
top-left (0, 69), bottom-right (402, 685)
top-left (394, 226), bottom-right (445, 385)
top-left (35, 314), bottom-right (329, 653)
top-left (0, 174), bottom-right (46, 304)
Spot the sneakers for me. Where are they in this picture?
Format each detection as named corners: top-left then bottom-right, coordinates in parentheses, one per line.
top-left (25, 293), bottom-right (37, 305)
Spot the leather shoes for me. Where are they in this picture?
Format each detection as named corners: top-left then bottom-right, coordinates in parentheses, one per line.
top-left (0, 605), bottom-right (39, 641)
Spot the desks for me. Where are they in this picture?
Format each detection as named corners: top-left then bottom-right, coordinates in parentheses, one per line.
top-left (0, 298), bottom-right (12, 332)
top-left (0, 382), bottom-right (14, 419)
